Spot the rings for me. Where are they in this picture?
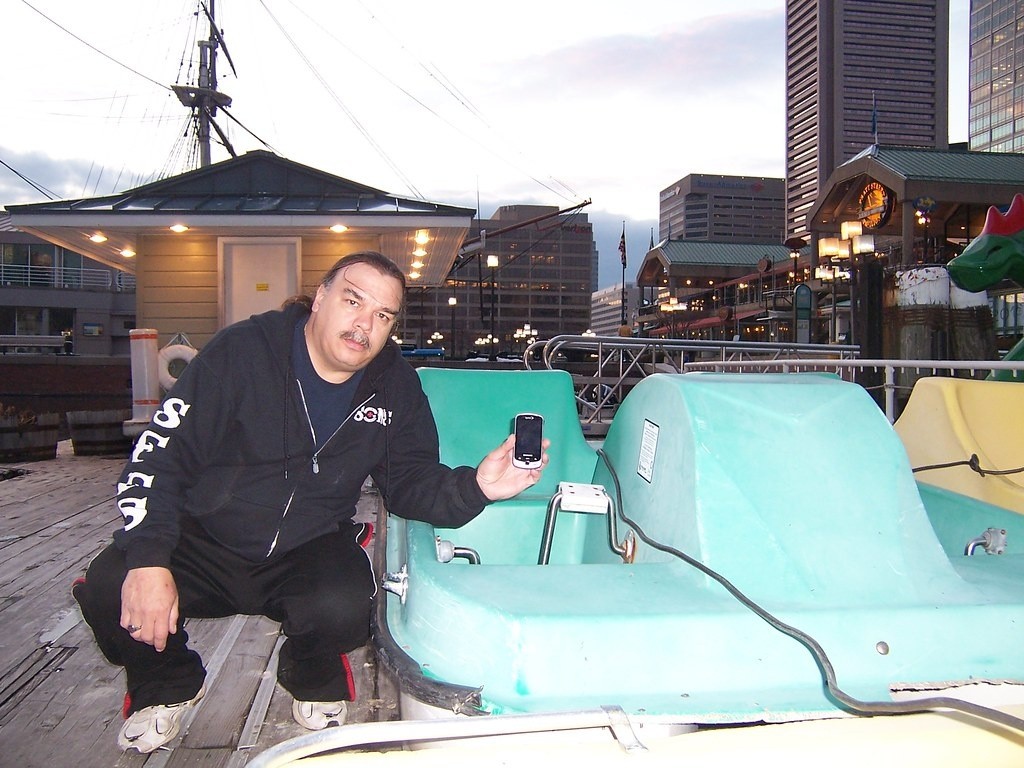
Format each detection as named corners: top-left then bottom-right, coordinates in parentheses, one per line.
top-left (128, 624), bottom-right (141, 633)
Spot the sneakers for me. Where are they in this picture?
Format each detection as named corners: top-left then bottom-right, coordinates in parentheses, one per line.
top-left (116, 678), bottom-right (207, 756)
top-left (276, 681), bottom-right (348, 731)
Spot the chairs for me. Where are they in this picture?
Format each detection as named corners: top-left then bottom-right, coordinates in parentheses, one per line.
top-left (894, 377), bottom-right (1024, 515)
top-left (414, 365), bottom-right (598, 563)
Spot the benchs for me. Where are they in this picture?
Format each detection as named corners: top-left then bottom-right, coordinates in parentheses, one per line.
top-left (0, 335), bottom-right (66, 354)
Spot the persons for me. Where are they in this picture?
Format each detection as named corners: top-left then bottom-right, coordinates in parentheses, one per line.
top-left (70, 251), bottom-right (550, 754)
top-left (618, 320), bottom-right (633, 338)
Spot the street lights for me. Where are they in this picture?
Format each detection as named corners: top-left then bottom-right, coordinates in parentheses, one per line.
top-left (389, 332), bottom-right (446, 350)
top-left (818, 221), bottom-right (876, 350)
top-left (448, 296), bottom-right (458, 358)
top-left (474, 323), bottom-right (599, 360)
top-left (661, 295), bottom-right (685, 342)
top-left (814, 265), bottom-right (849, 341)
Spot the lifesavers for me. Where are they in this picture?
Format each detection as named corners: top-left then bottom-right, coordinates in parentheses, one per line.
top-left (156, 345), bottom-right (200, 391)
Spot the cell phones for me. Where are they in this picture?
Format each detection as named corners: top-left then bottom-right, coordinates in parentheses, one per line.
top-left (512, 412), bottom-right (544, 469)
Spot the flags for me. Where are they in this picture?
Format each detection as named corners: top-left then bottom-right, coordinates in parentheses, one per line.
top-left (871, 95), bottom-right (877, 135)
top-left (617, 230), bottom-right (627, 269)
